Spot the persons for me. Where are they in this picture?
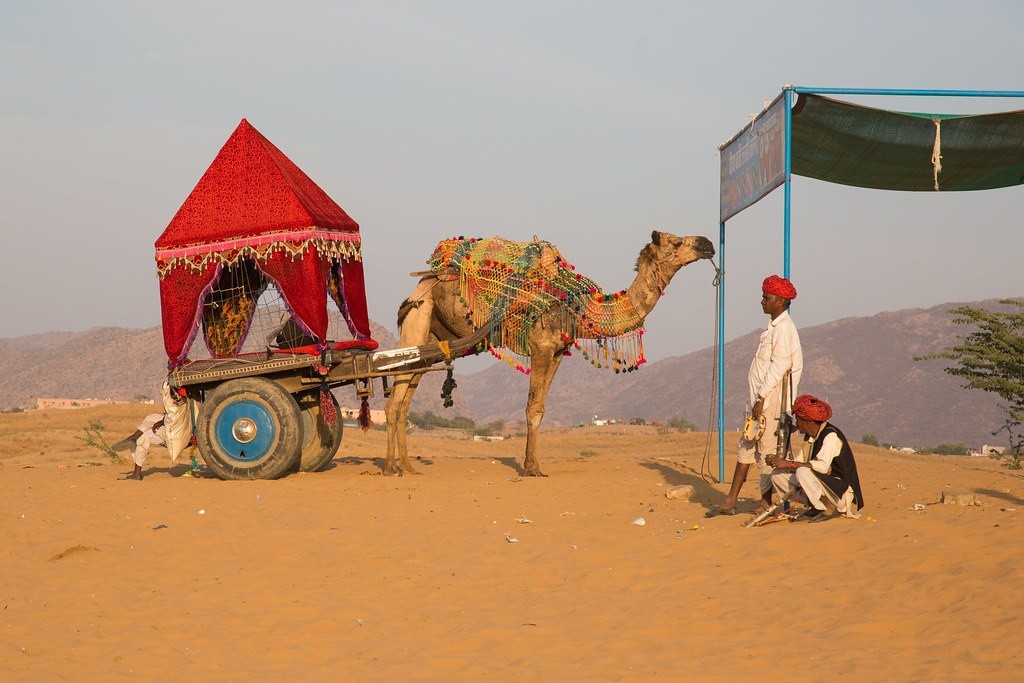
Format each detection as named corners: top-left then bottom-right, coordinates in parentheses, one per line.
top-left (765, 395), bottom-right (864, 522)
top-left (706, 275), bottom-right (804, 518)
top-left (111, 373), bottom-right (200, 481)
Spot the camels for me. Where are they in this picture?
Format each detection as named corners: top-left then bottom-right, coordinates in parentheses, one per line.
top-left (380, 228), bottom-right (716, 479)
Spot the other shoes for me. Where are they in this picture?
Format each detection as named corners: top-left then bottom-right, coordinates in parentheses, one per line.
top-left (111, 439), bottom-right (135, 452)
top-left (116, 475), bottom-right (142, 481)
top-left (809, 508), bottom-right (840, 523)
top-left (804, 508), bottom-right (819, 516)
top-left (705, 507), bottom-right (736, 516)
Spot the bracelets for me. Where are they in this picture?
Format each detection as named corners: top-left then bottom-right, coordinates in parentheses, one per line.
top-left (787, 460), bottom-right (796, 469)
top-left (755, 397), bottom-right (763, 403)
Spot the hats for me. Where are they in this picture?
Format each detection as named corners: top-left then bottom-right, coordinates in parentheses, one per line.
top-left (792, 394), bottom-right (833, 423)
top-left (762, 275), bottom-right (797, 300)
